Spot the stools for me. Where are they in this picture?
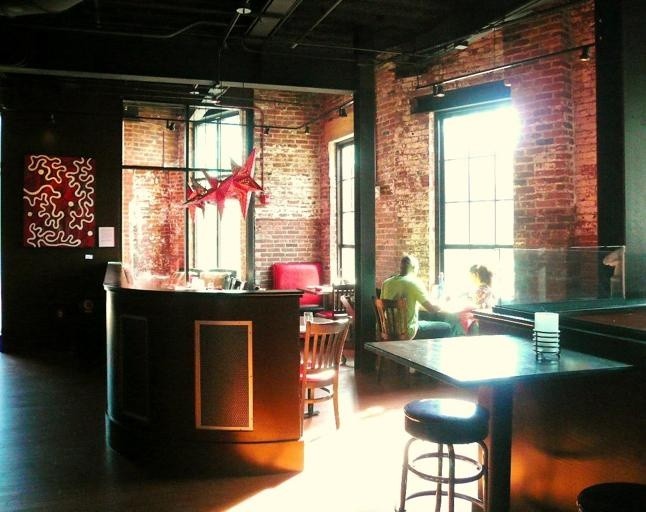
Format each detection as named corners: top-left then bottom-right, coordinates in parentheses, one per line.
top-left (399, 397), bottom-right (491, 511)
top-left (575, 479), bottom-right (646, 511)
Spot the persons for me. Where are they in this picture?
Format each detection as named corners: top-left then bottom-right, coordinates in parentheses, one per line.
top-left (456, 263), bottom-right (496, 333)
top-left (379, 254), bottom-right (453, 378)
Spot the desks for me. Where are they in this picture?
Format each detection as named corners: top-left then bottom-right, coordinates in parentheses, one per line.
top-left (363, 334), bottom-right (636, 511)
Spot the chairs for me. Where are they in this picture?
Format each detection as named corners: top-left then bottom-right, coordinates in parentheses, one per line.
top-left (269, 261), bottom-right (414, 430)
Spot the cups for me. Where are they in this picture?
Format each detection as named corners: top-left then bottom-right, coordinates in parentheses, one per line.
top-left (304, 311), bottom-right (313, 325)
